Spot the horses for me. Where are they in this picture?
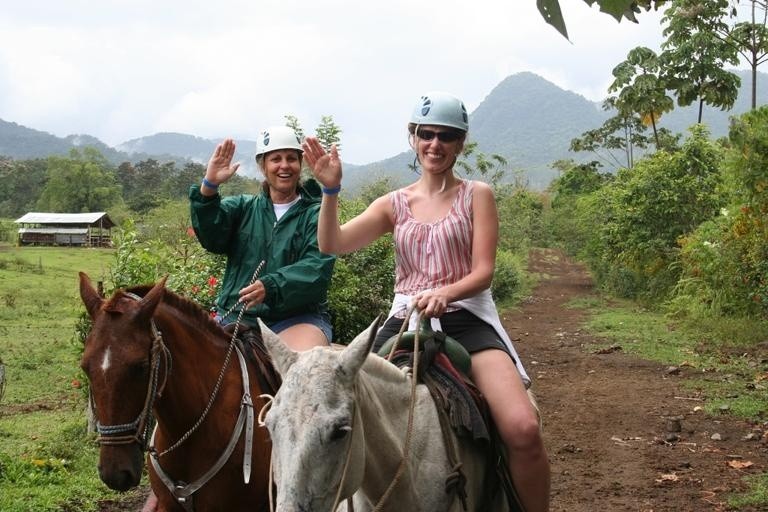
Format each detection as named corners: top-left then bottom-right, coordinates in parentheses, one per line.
top-left (255, 310), bottom-right (544, 512)
top-left (77, 270), bottom-right (348, 512)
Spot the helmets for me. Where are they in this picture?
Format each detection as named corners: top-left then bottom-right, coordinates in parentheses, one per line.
top-left (408, 91), bottom-right (470, 133)
top-left (255, 125), bottom-right (305, 156)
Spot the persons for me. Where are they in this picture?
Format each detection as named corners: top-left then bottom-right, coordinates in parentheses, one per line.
top-left (303, 91), bottom-right (551, 511)
top-left (142, 124), bottom-right (332, 512)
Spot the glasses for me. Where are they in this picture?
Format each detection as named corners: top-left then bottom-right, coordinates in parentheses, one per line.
top-left (416, 130), bottom-right (460, 142)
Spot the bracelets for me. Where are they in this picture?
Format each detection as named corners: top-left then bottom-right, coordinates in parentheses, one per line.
top-left (201, 177), bottom-right (223, 190)
top-left (321, 181), bottom-right (346, 197)
